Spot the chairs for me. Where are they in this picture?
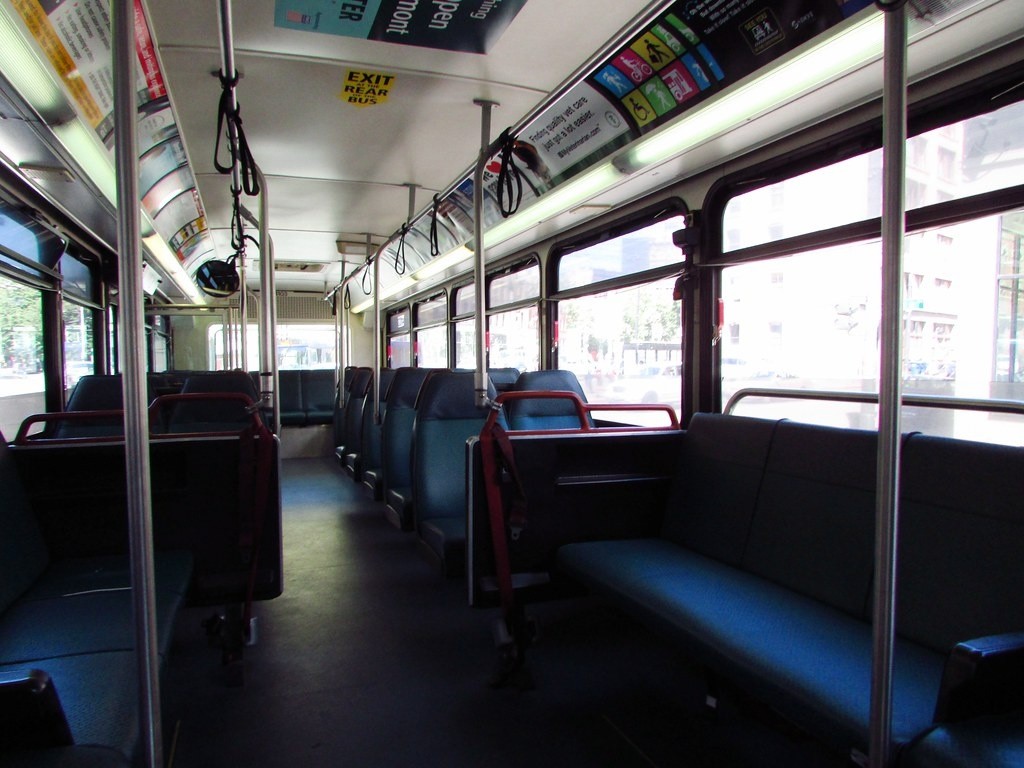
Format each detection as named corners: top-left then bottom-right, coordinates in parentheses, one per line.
top-left (0, 367), bottom-right (597, 768)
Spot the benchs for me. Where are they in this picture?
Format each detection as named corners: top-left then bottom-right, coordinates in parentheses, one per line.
top-left (555, 390), bottom-right (1024, 768)
